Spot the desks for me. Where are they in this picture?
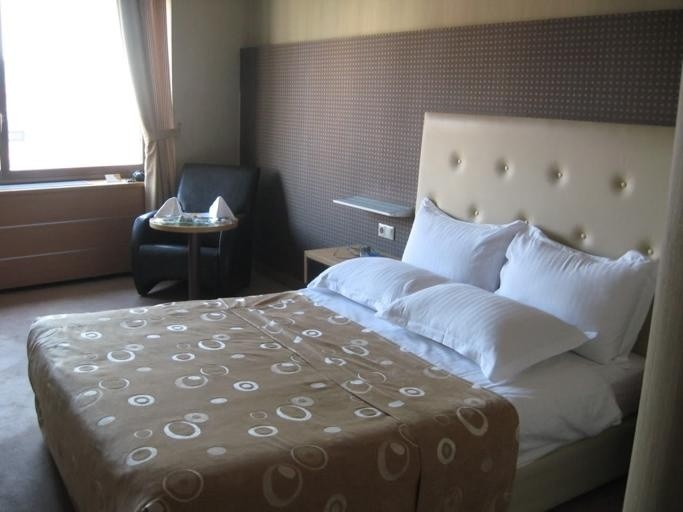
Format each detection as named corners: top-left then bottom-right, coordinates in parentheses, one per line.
top-left (148, 211), bottom-right (239, 303)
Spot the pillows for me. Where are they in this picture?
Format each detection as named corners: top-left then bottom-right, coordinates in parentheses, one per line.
top-left (493, 221), bottom-right (653, 365)
top-left (307, 257), bottom-right (450, 313)
top-left (401, 197), bottom-right (527, 293)
top-left (375, 283), bottom-right (599, 383)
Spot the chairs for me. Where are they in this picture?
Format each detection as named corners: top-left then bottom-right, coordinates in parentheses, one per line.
top-left (129, 162), bottom-right (261, 300)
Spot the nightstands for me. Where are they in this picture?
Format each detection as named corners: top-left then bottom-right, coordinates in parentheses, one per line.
top-left (304, 244), bottom-right (401, 287)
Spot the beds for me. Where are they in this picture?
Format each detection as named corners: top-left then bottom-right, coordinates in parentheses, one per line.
top-left (26, 110), bottom-right (674, 512)
